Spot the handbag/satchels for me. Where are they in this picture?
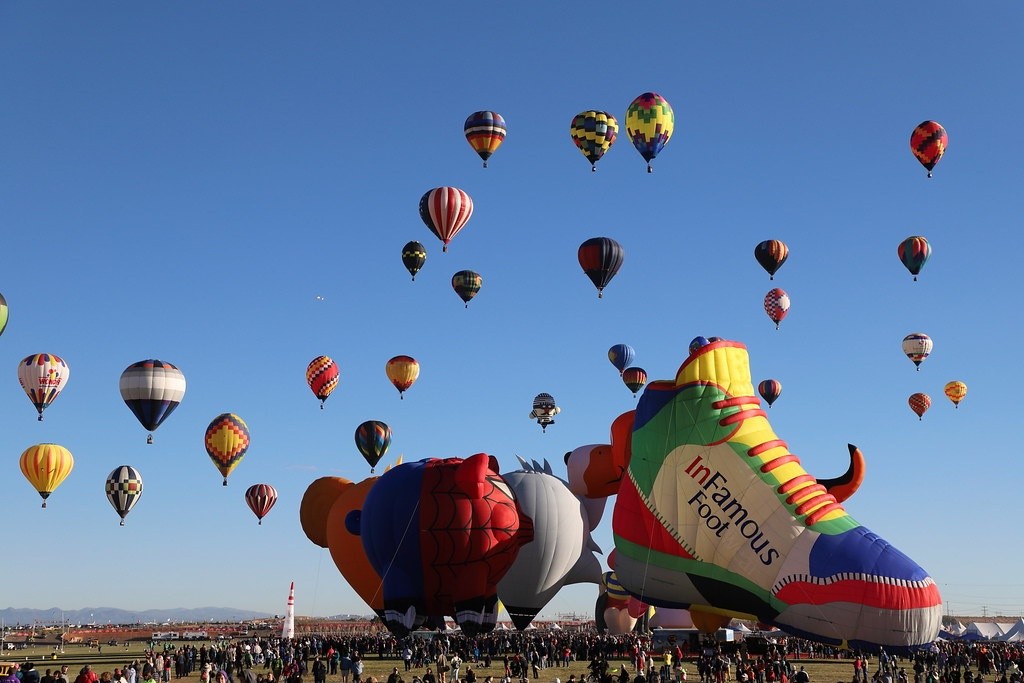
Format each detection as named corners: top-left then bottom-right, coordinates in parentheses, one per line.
top-left (441, 666), bottom-right (450, 673)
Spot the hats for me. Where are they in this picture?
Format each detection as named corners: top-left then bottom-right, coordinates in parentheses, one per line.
top-left (215, 670), bottom-right (228, 682)
top-left (85, 665), bottom-right (94, 669)
top-left (8, 668), bottom-right (16, 674)
top-left (204, 663), bottom-right (209, 668)
top-left (23, 662), bottom-right (34, 671)
top-left (14, 663), bottom-right (19, 669)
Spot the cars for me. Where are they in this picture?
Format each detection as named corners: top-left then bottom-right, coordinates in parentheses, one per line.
top-left (746, 636), bottom-right (788, 655)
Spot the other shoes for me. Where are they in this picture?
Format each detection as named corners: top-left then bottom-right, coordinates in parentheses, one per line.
top-left (563, 667), bottom-right (565, 668)
top-left (729, 680), bottom-right (731, 682)
top-left (567, 667), bottom-right (569, 668)
top-left (700, 681), bottom-right (703, 683)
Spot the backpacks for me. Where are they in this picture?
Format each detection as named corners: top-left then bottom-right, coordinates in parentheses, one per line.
top-left (680, 672), bottom-right (686, 681)
top-left (453, 657), bottom-right (461, 670)
top-left (201, 669), bottom-right (208, 680)
top-left (770, 672), bottom-right (776, 681)
top-left (166, 658), bottom-right (171, 668)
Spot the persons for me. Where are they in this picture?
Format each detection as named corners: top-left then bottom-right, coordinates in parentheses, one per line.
top-left (0, 627), bottom-right (1024, 683)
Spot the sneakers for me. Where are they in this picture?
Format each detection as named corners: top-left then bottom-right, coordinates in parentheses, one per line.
top-left (612, 342), bottom-right (942, 651)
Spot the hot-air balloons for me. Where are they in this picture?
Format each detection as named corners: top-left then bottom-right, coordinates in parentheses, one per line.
top-left (299, 335), bottom-right (942, 654)
top-left (18, 354), bottom-right (69, 422)
top-left (624, 92), bottom-right (675, 173)
top-left (0, 292), bottom-right (9, 337)
top-left (944, 381), bottom-right (967, 410)
top-left (401, 186), bottom-right (482, 308)
top-left (119, 360), bottom-right (186, 444)
top-left (244, 484), bottom-right (277, 524)
top-left (578, 236), bottom-right (625, 299)
top-left (20, 444), bottom-right (74, 508)
top-left (106, 465), bottom-right (143, 526)
top-left (909, 120), bottom-right (948, 180)
top-left (754, 239), bottom-right (789, 282)
top-left (897, 236), bottom-right (932, 283)
top-left (204, 412), bottom-right (249, 486)
top-left (463, 110), bottom-right (507, 167)
top-left (570, 110), bottom-right (620, 173)
top-left (763, 287), bottom-right (791, 331)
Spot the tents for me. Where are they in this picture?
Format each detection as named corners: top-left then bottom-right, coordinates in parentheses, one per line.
top-left (730, 616), bottom-right (1024, 642)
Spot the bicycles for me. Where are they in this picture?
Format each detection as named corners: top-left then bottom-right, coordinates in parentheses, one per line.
top-left (588, 667), bottom-right (620, 683)
